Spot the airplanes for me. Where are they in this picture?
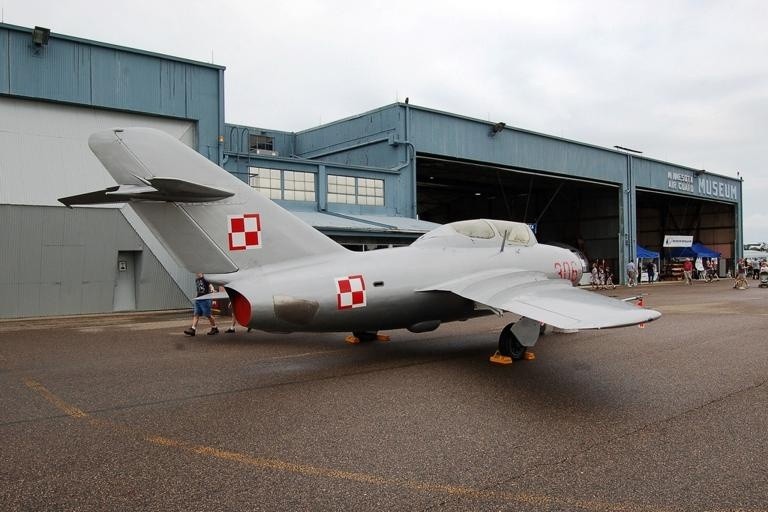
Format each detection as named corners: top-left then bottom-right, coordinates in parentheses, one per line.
top-left (58, 127), bottom-right (661, 360)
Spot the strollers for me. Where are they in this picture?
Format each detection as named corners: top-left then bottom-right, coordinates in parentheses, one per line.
top-left (758, 261), bottom-right (768, 288)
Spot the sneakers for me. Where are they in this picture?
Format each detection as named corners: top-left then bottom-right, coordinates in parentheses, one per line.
top-left (225, 328), bottom-right (235, 332)
top-left (184, 328), bottom-right (195, 336)
top-left (207, 327), bottom-right (219, 335)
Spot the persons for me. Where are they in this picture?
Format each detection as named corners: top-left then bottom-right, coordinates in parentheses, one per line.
top-left (624, 256), bottom-right (720, 288)
top-left (588, 257), bottom-right (616, 290)
top-left (183, 271), bottom-right (219, 337)
top-left (732, 256), bottom-right (768, 289)
top-left (223, 301), bottom-right (251, 333)
